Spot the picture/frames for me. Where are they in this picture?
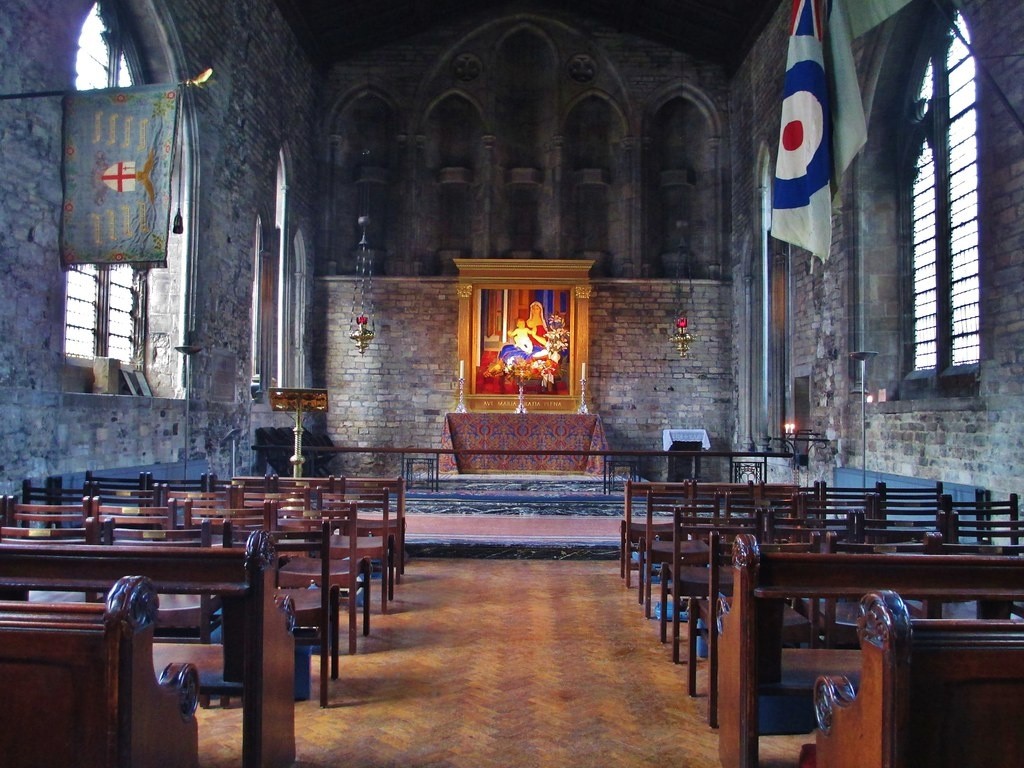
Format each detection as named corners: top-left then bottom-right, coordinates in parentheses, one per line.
top-left (119, 370), bottom-right (139, 396)
top-left (132, 370), bottom-right (154, 396)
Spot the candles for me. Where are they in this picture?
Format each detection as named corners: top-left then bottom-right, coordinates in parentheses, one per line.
top-left (459, 360), bottom-right (464, 380)
top-left (581, 363), bottom-right (586, 380)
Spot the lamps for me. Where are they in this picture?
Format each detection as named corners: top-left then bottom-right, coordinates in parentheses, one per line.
top-left (667, 237), bottom-right (696, 357)
top-left (350, 144), bottom-right (374, 353)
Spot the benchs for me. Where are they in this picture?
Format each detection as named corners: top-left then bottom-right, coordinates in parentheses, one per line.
top-left (619, 481), bottom-right (1024, 768)
top-left (0, 474), bottom-right (411, 768)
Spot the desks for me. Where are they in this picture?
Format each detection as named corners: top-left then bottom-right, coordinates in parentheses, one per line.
top-left (438, 412), bottom-right (612, 477)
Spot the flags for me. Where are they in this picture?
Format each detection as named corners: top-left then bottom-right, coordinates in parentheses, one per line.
top-left (763, 0), bottom-right (868, 265)
top-left (58, 83), bottom-right (180, 272)
